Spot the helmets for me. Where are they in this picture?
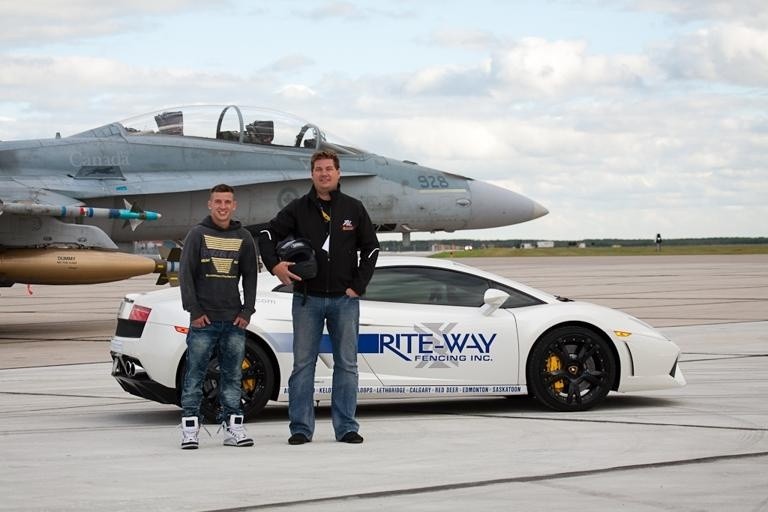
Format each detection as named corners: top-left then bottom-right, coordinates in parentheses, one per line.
top-left (277, 238), bottom-right (318, 280)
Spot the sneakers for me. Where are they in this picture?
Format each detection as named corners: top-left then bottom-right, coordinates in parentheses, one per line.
top-left (180, 416), bottom-right (200, 449)
top-left (222, 414), bottom-right (254, 447)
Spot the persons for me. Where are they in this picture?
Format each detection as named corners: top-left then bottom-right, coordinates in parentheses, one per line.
top-left (655, 233), bottom-right (662, 251)
top-left (259, 150), bottom-right (380, 442)
top-left (178, 185), bottom-right (257, 448)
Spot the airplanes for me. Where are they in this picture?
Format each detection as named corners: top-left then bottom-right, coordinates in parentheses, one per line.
top-left (0, 99), bottom-right (550, 289)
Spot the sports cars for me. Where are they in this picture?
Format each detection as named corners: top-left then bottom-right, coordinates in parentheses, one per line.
top-left (110, 250), bottom-right (688, 410)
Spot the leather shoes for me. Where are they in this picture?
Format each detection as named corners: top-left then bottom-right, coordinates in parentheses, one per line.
top-left (288, 433), bottom-right (310, 445)
top-left (339, 431), bottom-right (363, 443)
